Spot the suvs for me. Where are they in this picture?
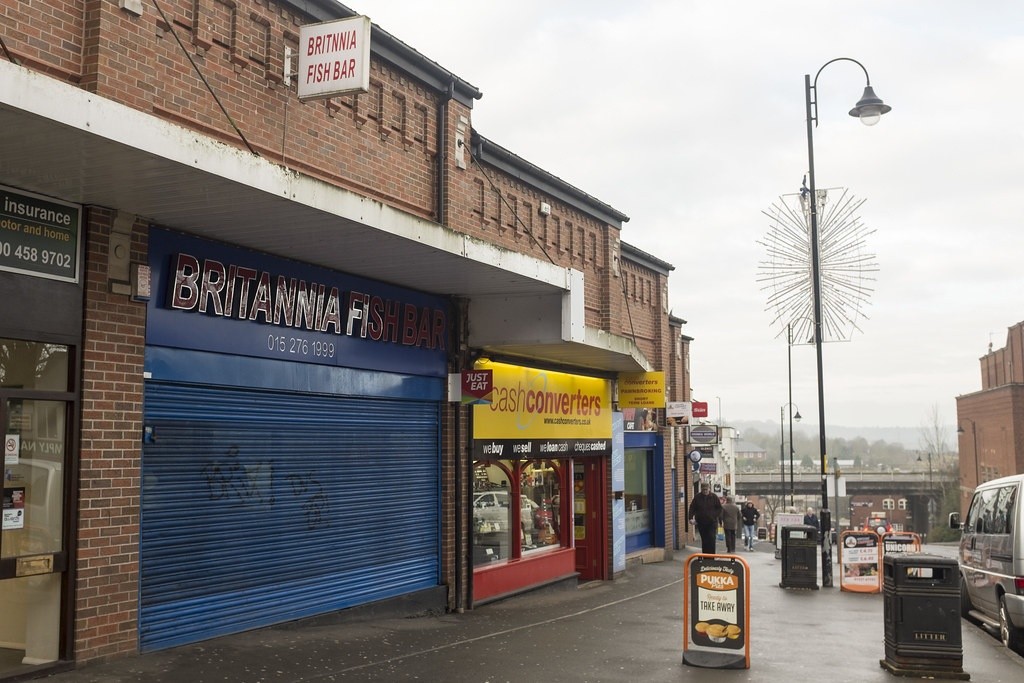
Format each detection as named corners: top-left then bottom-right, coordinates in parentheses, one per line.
top-left (818, 520), bottom-right (842, 544)
top-left (864, 516), bottom-right (892, 535)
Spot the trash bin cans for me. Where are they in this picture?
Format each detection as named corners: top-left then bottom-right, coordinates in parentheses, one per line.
top-left (779, 524), bottom-right (819, 591)
top-left (879, 553), bottom-right (971, 680)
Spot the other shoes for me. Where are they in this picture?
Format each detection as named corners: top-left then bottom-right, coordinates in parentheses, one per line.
top-left (744, 546), bottom-right (748, 552)
top-left (749, 547), bottom-right (753, 551)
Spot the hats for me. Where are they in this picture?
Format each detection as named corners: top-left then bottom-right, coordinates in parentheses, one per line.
top-left (701, 483), bottom-right (709, 490)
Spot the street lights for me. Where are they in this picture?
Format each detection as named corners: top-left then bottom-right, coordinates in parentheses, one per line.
top-left (803, 57), bottom-right (892, 588)
top-left (917, 450), bottom-right (934, 529)
top-left (957, 417), bottom-right (979, 487)
top-left (788, 316), bottom-right (817, 514)
top-left (781, 403), bottom-right (802, 513)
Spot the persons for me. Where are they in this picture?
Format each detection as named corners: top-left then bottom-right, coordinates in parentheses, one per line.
top-left (688, 482), bottom-right (721, 560)
top-left (804, 507), bottom-right (819, 540)
top-left (742, 502), bottom-right (760, 551)
top-left (719, 496), bottom-right (741, 554)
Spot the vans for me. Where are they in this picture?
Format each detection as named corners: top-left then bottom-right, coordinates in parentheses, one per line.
top-left (948, 474), bottom-right (1024, 649)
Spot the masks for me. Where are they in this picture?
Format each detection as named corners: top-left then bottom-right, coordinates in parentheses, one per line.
top-left (747, 504), bottom-right (752, 508)
